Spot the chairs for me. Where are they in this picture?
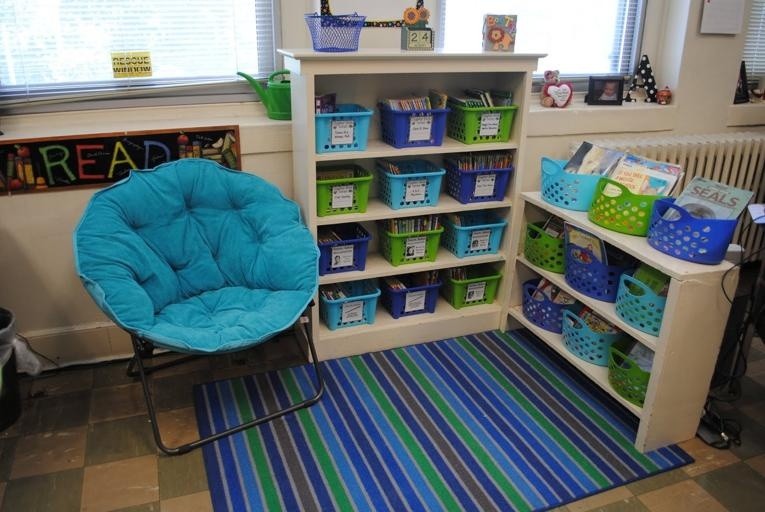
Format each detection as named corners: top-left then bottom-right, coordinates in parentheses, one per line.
top-left (72, 158), bottom-right (323, 456)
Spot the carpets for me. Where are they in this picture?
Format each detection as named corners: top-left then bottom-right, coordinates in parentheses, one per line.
top-left (192, 329), bottom-right (694, 512)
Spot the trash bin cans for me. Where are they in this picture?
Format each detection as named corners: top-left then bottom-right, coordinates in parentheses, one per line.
top-left (0, 307), bottom-right (22, 432)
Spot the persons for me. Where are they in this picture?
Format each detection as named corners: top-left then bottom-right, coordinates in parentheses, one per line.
top-left (598, 80), bottom-right (618, 101)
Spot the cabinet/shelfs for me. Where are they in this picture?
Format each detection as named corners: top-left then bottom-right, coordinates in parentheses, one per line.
top-left (500, 192), bottom-right (740, 455)
top-left (277, 48), bottom-right (548, 363)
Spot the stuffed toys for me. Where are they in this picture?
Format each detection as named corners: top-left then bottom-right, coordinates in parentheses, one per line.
top-left (541, 70), bottom-right (573, 107)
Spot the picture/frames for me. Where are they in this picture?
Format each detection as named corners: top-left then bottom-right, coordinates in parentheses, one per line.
top-left (589, 76), bottom-right (624, 105)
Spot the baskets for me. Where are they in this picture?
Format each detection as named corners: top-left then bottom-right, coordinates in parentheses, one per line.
top-left (318, 222), bottom-right (372, 276)
top-left (319, 282), bottom-right (383, 332)
top-left (646, 194), bottom-right (739, 266)
top-left (314, 102), bottom-right (374, 153)
top-left (539, 156), bottom-right (601, 212)
top-left (442, 156), bottom-right (514, 204)
top-left (445, 211), bottom-right (508, 259)
top-left (305, 14), bottom-right (367, 53)
top-left (381, 272), bottom-right (442, 320)
top-left (521, 220), bottom-right (668, 408)
top-left (443, 268), bottom-right (504, 310)
top-left (588, 171), bottom-right (672, 237)
top-left (378, 159), bottom-right (446, 210)
top-left (378, 102), bottom-right (452, 149)
top-left (315, 164), bottom-right (374, 217)
top-left (376, 218), bottom-right (444, 266)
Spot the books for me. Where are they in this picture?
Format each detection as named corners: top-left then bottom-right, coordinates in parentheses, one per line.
top-left (618, 265), bottom-right (668, 337)
top-left (317, 164), bottom-right (354, 181)
top-left (527, 277), bottom-right (576, 332)
top-left (560, 219), bottom-right (609, 299)
top-left (450, 153), bottom-right (512, 171)
top-left (532, 216), bottom-right (568, 273)
top-left (444, 213), bottom-right (461, 226)
top-left (315, 92), bottom-right (337, 115)
top-left (542, 142), bottom-right (622, 206)
top-left (380, 91), bottom-right (447, 144)
top-left (612, 338), bottom-right (655, 406)
top-left (564, 303), bottom-right (621, 365)
top-left (318, 283), bottom-right (347, 301)
top-left (450, 91), bottom-right (512, 108)
top-left (377, 215), bottom-right (438, 234)
top-left (594, 153), bottom-right (683, 230)
top-left (380, 160), bottom-right (400, 175)
top-left (317, 228), bottom-right (355, 268)
top-left (657, 174), bottom-right (754, 257)
top-left (385, 266), bottom-right (467, 291)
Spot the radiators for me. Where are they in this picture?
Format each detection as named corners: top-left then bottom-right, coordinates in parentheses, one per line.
top-left (567, 130), bottom-right (765, 264)
